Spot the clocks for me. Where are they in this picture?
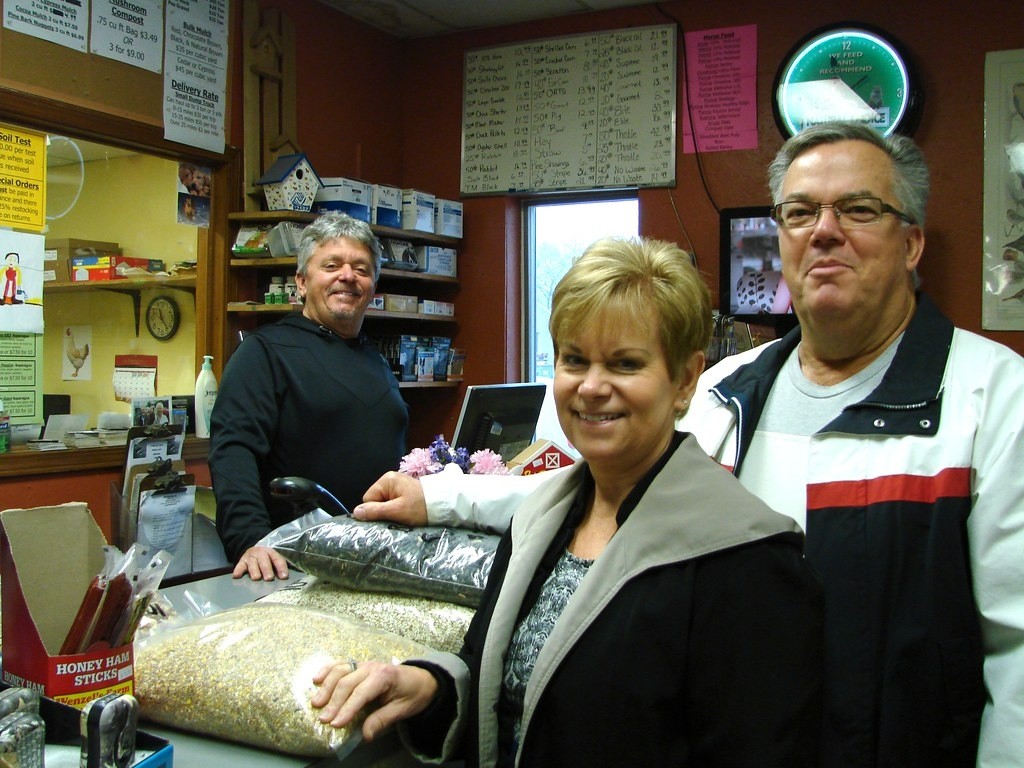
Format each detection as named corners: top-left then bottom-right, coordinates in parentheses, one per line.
top-left (777, 29), bottom-right (909, 145)
top-left (148, 298), bottom-right (176, 338)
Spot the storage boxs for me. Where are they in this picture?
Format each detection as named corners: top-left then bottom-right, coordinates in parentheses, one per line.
top-left (402, 190), bottom-right (435, 233)
top-left (385, 295), bottom-right (418, 313)
top-left (417, 246), bottom-right (457, 278)
top-left (434, 199), bottom-right (464, 239)
top-left (383, 335), bottom-right (464, 383)
top-left (366, 293), bottom-right (383, 311)
top-left (0, 501), bottom-right (136, 712)
top-left (419, 300), bottom-right (456, 317)
top-left (385, 238), bottom-right (418, 271)
top-left (266, 221), bottom-right (306, 259)
top-left (231, 223), bottom-right (272, 259)
top-left (311, 177), bottom-right (371, 222)
top-left (44, 238), bottom-right (118, 283)
top-left (72, 256), bottom-right (164, 283)
top-left (371, 184), bottom-right (402, 227)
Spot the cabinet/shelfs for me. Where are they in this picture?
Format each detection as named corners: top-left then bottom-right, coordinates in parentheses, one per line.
top-left (224, 208), bottom-right (466, 390)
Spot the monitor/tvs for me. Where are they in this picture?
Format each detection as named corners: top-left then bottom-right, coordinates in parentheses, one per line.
top-left (720, 204), bottom-right (800, 327)
top-left (450, 383), bottom-right (547, 466)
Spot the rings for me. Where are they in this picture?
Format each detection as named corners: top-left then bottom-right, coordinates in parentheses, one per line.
top-left (348, 657), bottom-right (357, 672)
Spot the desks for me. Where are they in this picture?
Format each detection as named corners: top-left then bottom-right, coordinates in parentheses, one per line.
top-left (139, 568), bottom-right (311, 768)
top-left (0, 432), bottom-right (213, 545)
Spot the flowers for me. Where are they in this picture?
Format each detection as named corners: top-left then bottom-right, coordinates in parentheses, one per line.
top-left (397, 433), bottom-right (514, 477)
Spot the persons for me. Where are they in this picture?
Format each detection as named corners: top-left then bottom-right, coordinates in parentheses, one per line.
top-left (354, 122), bottom-right (1024, 768)
top-left (136, 403), bottom-right (169, 425)
top-left (208, 215), bottom-right (410, 581)
top-left (311, 240), bottom-right (828, 768)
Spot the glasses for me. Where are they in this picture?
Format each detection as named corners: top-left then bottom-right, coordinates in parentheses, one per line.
top-left (771, 196), bottom-right (914, 226)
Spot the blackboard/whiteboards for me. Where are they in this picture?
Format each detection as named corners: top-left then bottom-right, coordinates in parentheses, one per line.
top-left (459, 23), bottom-right (678, 197)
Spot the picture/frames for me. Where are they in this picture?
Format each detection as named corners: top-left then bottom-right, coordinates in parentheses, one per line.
top-left (131, 395), bottom-right (173, 428)
top-left (981, 49), bottom-right (1024, 331)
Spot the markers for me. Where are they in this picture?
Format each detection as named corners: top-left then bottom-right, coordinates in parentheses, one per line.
top-left (594, 184), bottom-right (639, 189)
top-left (556, 186), bottom-right (592, 191)
top-left (509, 187), bottom-right (539, 192)
